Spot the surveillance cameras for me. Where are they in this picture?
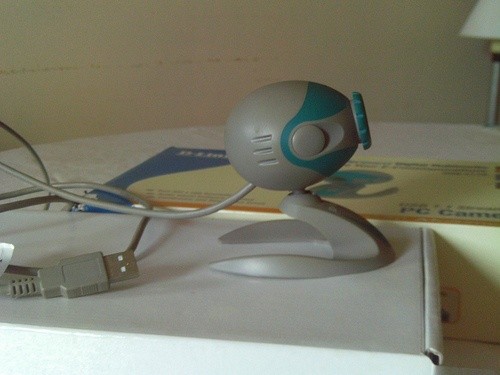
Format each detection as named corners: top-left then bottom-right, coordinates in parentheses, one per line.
top-left (207, 80), bottom-right (397, 279)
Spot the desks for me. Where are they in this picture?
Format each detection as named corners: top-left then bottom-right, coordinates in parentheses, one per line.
top-left (0, 120), bottom-right (500, 374)
